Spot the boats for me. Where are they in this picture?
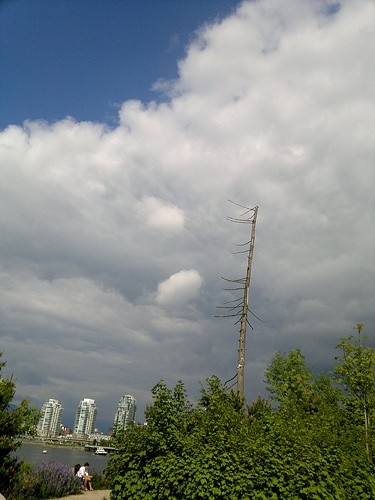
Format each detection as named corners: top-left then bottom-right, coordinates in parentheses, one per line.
top-left (42, 447), bottom-right (47, 454)
top-left (95, 447), bottom-right (107, 455)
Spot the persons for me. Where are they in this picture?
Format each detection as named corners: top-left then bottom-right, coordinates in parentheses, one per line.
top-left (75, 462), bottom-right (94, 491)
top-left (72, 464), bottom-right (82, 478)
